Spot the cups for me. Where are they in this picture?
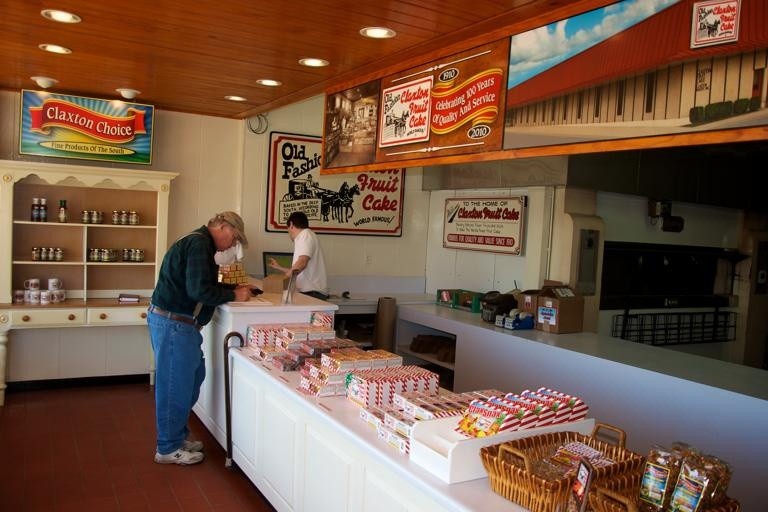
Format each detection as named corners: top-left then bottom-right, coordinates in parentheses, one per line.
top-left (23, 278), bottom-right (41, 290)
top-left (52, 291), bottom-right (65, 304)
top-left (29, 290), bottom-right (40, 304)
top-left (23, 290), bottom-right (30, 303)
top-left (40, 290), bottom-right (51, 304)
top-left (48, 279), bottom-right (63, 291)
top-left (12, 290), bottom-right (24, 303)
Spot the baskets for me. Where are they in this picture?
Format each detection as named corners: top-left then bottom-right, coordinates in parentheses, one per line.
top-left (567, 469), bottom-right (730, 512)
top-left (479, 422), bottom-right (646, 512)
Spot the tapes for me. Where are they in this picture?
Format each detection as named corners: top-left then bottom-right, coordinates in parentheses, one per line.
top-left (519, 312), bottom-right (528, 321)
top-left (509, 308), bottom-right (522, 320)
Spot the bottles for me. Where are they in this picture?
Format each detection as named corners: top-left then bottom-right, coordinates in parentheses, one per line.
top-left (110, 249), bottom-right (117, 261)
top-left (98, 211), bottom-right (104, 223)
top-left (90, 249), bottom-right (95, 261)
top-left (128, 249), bottom-right (132, 260)
top-left (123, 248), bottom-right (128, 260)
top-left (130, 211), bottom-right (138, 223)
top-left (104, 249), bottom-right (110, 261)
top-left (54, 247), bottom-right (63, 260)
top-left (120, 211), bottom-right (129, 224)
top-left (31, 248), bottom-right (40, 260)
top-left (97, 248), bottom-right (101, 261)
top-left (131, 249), bottom-right (136, 261)
top-left (82, 211), bottom-right (90, 223)
top-left (39, 199), bottom-right (47, 222)
top-left (92, 211), bottom-right (98, 223)
top-left (49, 248), bottom-right (54, 260)
top-left (141, 249), bottom-right (144, 262)
top-left (40, 247), bottom-right (47, 260)
top-left (58, 200), bottom-right (67, 223)
top-left (94, 249), bottom-right (100, 261)
top-left (113, 211), bottom-right (119, 224)
top-left (136, 250), bottom-right (141, 262)
top-left (101, 248), bottom-right (105, 262)
top-left (31, 198), bottom-right (40, 222)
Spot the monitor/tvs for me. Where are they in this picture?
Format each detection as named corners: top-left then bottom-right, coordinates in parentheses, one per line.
top-left (262, 252), bottom-right (294, 278)
top-left (599, 241), bottom-right (739, 311)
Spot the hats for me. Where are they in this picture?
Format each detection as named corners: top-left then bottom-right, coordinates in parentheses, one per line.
top-left (217, 210), bottom-right (248, 249)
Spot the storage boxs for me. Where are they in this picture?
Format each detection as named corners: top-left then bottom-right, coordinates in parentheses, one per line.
top-left (536, 285), bottom-right (584, 334)
top-left (0, 159), bottom-right (181, 408)
top-left (517, 290), bottom-right (540, 330)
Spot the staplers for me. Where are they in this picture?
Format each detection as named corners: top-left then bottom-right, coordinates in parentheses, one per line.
top-left (341, 291), bottom-right (351, 301)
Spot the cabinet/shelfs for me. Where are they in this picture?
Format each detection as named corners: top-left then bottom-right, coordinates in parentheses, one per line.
top-left (171, 271), bottom-right (765, 507)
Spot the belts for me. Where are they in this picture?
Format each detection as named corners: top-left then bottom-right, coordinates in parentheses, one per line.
top-left (148, 306), bottom-right (203, 330)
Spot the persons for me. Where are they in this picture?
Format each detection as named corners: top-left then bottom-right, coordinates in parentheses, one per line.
top-left (147, 212), bottom-right (258, 465)
top-left (269, 211), bottom-right (328, 299)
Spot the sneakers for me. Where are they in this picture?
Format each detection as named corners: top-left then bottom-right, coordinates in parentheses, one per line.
top-left (156, 439), bottom-right (203, 452)
top-left (153, 449), bottom-right (204, 466)
